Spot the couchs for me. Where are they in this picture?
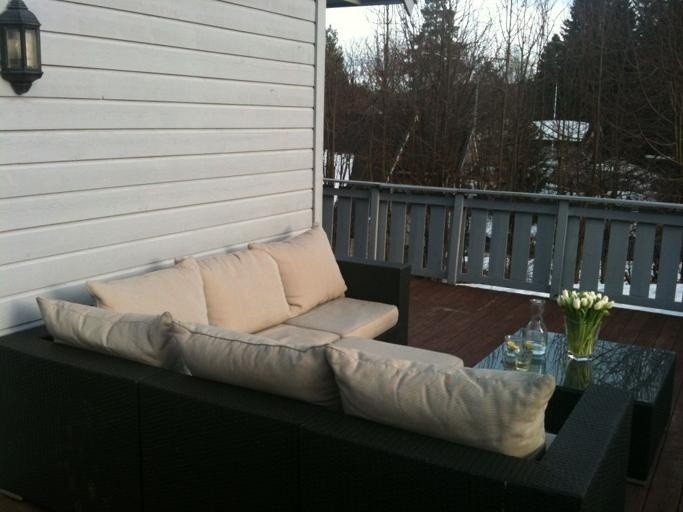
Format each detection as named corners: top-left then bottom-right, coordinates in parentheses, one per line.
top-left (1, 225), bottom-right (638, 512)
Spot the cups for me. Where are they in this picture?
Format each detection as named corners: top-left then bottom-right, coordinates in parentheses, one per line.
top-left (500, 334), bottom-right (532, 372)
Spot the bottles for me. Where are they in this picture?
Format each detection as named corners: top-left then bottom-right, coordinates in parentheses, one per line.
top-left (523, 298), bottom-right (548, 357)
top-left (529, 356), bottom-right (547, 378)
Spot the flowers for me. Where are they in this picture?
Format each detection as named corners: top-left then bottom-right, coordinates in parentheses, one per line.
top-left (553, 289), bottom-right (615, 354)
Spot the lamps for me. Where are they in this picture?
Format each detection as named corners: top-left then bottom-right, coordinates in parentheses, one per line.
top-left (0, 0), bottom-right (45, 98)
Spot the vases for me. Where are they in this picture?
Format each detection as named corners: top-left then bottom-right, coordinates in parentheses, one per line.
top-left (563, 315), bottom-right (602, 362)
